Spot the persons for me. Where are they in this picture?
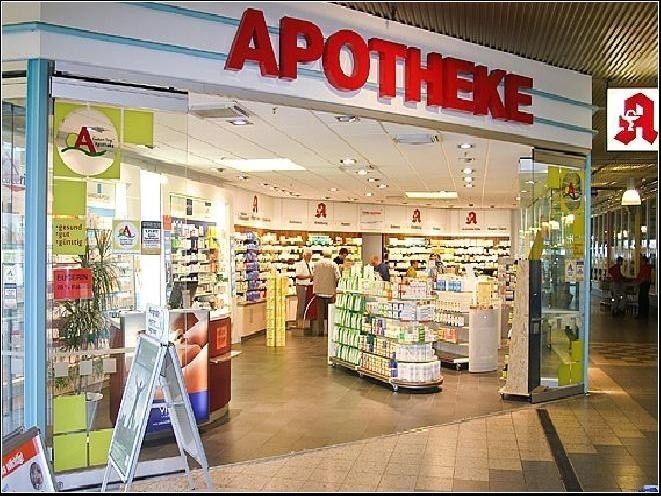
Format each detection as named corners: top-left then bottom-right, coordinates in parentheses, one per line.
top-left (406, 260), bottom-right (418, 277)
top-left (631, 255), bottom-right (652, 320)
top-left (335, 247), bottom-right (349, 263)
top-left (313, 248), bottom-right (340, 338)
top-left (608, 257), bottom-right (627, 316)
top-left (154, 312), bottom-right (208, 401)
top-left (434, 254), bottom-right (440, 261)
top-left (295, 247), bottom-right (314, 322)
top-left (369, 255), bottom-right (390, 282)
top-left (430, 254), bottom-right (435, 260)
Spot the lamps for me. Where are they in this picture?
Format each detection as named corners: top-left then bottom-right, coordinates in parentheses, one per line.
top-left (621, 164), bottom-right (642, 207)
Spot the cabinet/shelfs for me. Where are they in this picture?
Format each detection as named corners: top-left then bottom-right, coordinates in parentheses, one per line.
top-left (328, 287), bottom-right (444, 389)
top-left (169, 218), bottom-right (363, 349)
top-left (384, 233), bottom-right (544, 400)
